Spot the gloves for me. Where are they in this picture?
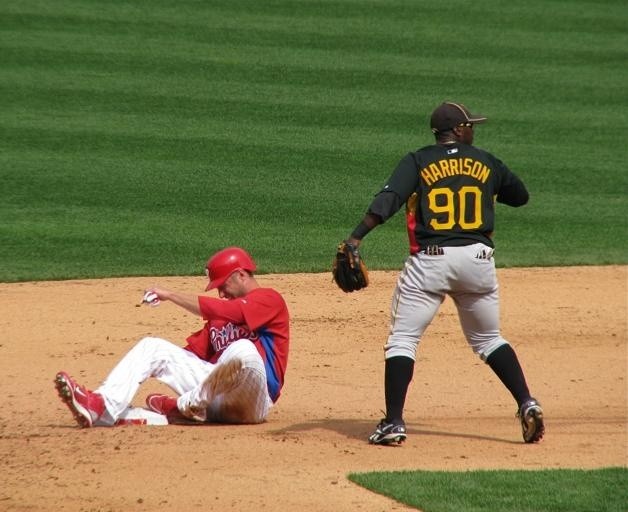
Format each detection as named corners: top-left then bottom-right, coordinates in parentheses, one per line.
top-left (140, 288), bottom-right (160, 306)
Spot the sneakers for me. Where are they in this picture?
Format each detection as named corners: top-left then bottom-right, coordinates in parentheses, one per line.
top-left (146, 394), bottom-right (182, 417)
top-left (515, 399), bottom-right (545, 443)
top-left (368, 419), bottom-right (407, 445)
top-left (54, 372), bottom-right (106, 428)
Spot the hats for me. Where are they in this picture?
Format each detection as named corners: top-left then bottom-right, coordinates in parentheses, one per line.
top-left (431, 102), bottom-right (488, 134)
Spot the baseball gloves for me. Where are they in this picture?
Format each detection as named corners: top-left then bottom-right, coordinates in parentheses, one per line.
top-left (330, 240), bottom-right (369, 293)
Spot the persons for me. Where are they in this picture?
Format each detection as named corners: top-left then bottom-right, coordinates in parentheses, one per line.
top-left (53, 245), bottom-right (290, 429)
top-left (330, 101), bottom-right (546, 447)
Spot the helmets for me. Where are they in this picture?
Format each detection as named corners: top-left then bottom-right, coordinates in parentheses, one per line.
top-left (205, 247), bottom-right (256, 292)
